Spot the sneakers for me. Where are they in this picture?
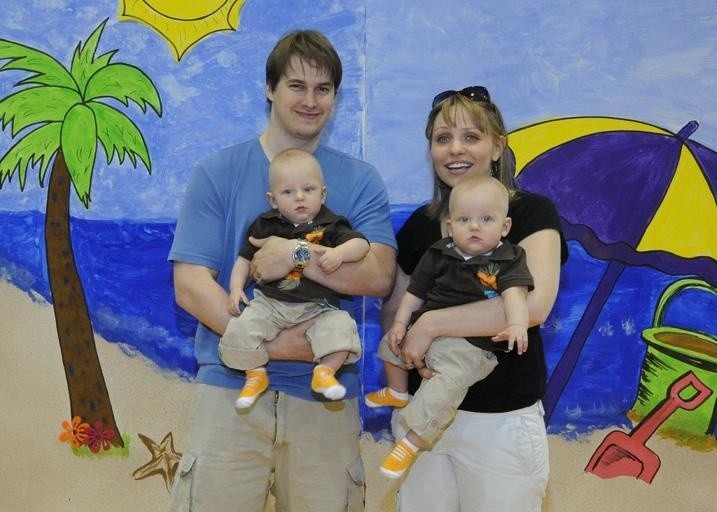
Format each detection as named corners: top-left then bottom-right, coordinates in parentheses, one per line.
top-left (361, 385), bottom-right (410, 409)
top-left (230, 366), bottom-right (272, 412)
top-left (306, 362), bottom-right (348, 401)
top-left (377, 436), bottom-right (420, 480)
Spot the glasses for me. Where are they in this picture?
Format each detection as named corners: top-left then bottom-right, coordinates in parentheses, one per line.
top-left (428, 81), bottom-right (492, 111)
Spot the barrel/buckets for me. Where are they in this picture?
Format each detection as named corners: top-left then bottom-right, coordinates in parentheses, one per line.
top-left (627, 274), bottom-right (717, 439)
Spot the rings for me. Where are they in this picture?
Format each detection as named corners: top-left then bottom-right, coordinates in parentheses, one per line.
top-left (402, 360), bottom-right (414, 368)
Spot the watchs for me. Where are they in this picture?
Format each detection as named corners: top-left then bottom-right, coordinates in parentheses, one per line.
top-left (293, 238), bottom-right (312, 274)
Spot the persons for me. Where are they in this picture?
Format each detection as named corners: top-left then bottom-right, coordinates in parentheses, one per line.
top-left (217, 146), bottom-right (371, 412)
top-left (361, 173), bottom-right (537, 478)
top-left (379, 84), bottom-right (571, 512)
top-left (165, 28), bottom-right (403, 512)
